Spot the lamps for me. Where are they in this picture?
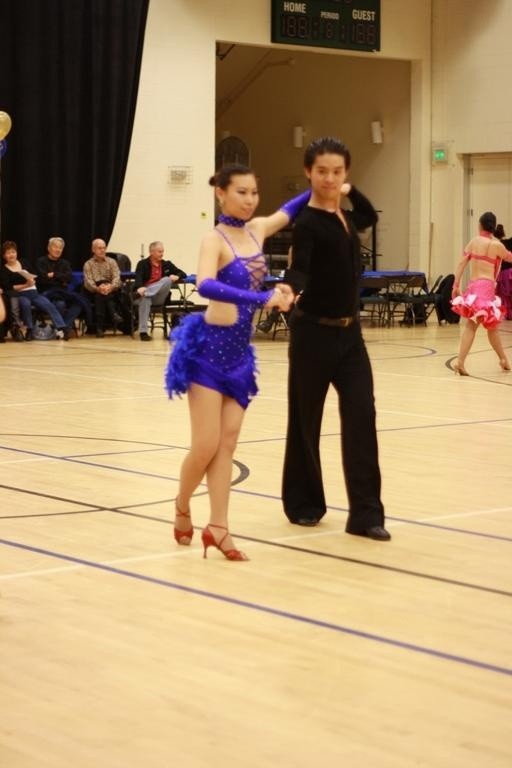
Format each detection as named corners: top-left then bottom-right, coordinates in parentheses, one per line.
top-left (371, 120), bottom-right (383, 144)
top-left (293, 126), bottom-right (306, 148)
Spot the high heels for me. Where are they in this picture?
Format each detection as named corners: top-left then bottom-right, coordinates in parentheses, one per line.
top-left (174, 497), bottom-right (193, 545)
top-left (453, 365), bottom-right (470, 375)
top-left (500, 359), bottom-right (511, 369)
top-left (202, 523), bottom-right (248, 561)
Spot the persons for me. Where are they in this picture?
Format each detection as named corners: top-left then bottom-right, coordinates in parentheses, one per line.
top-left (275, 139), bottom-right (391, 541)
top-left (30, 237), bottom-right (82, 341)
top-left (129, 241), bottom-right (187, 341)
top-left (255, 246), bottom-right (293, 333)
top-left (494, 224), bottom-right (512, 320)
top-left (0, 241), bottom-right (75, 341)
top-left (83, 238), bottom-right (125, 337)
top-left (165, 164), bottom-right (313, 561)
top-left (450, 212), bottom-right (512, 376)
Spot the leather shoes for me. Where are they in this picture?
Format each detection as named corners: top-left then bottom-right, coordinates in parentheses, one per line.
top-left (140, 332), bottom-right (152, 340)
top-left (14, 312), bottom-right (123, 341)
top-left (256, 324), bottom-right (268, 333)
top-left (297, 517), bottom-right (317, 525)
top-left (132, 290), bottom-right (141, 300)
top-left (361, 525), bottom-right (391, 540)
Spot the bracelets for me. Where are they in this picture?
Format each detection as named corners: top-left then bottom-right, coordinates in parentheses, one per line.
top-left (453, 282), bottom-right (460, 288)
